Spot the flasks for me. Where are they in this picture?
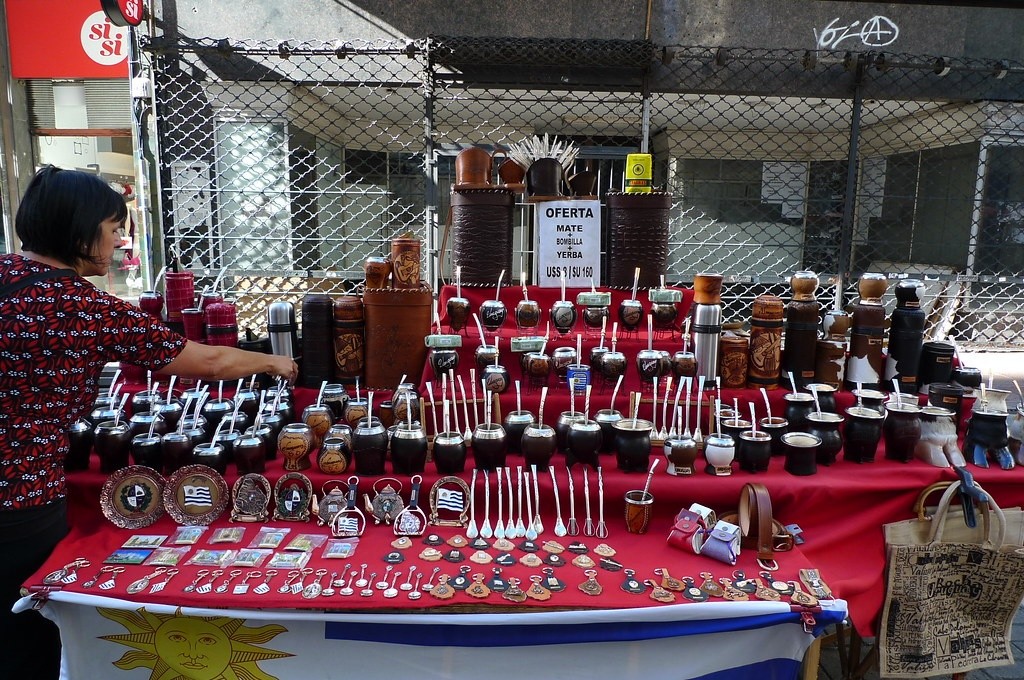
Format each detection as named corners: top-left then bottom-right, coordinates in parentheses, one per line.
top-left (780, 301), bottom-right (819, 390)
top-left (746, 295), bottom-right (783, 390)
top-left (333, 296), bottom-right (364, 389)
top-left (845, 304), bottom-right (886, 391)
top-left (267, 302), bottom-right (300, 388)
top-left (301, 295), bottom-right (334, 388)
top-left (205, 303), bottom-right (238, 387)
top-left (884, 307), bottom-right (925, 393)
top-left (692, 303), bottom-right (722, 390)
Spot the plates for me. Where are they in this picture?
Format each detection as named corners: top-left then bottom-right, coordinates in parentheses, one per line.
top-left (100, 466), bottom-right (168, 529)
top-left (163, 464), bottom-right (228, 526)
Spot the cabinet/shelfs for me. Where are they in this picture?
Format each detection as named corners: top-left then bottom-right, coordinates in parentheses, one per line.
top-left (69, 287), bottom-right (1023, 529)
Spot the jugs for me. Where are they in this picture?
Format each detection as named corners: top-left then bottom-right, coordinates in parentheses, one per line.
top-left (454, 147), bottom-right (507, 185)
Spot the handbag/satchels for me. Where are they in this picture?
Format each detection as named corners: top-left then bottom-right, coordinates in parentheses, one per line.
top-left (882, 481), bottom-right (1024, 676)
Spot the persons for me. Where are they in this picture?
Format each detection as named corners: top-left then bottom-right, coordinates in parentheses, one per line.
top-left (0, 167), bottom-right (299, 680)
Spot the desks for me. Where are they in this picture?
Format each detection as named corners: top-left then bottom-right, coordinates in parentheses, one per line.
top-left (17, 527), bottom-right (846, 680)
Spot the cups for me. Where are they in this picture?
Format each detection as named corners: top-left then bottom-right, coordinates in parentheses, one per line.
top-left (364, 238), bottom-right (420, 290)
top-left (428, 296), bottom-right (698, 394)
top-left (139, 273), bottom-right (222, 342)
top-left (664, 437), bottom-right (697, 475)
top-left (558, 408), bottom-right (656, 470)
top-left (624, 490), bottom-right (654, 534)
top-left (240, 337), bottom-right (269, 389)
top-left (705, 336), bottom-right (1024, 476)
top-left (432, 410), bottom-right (555, 473)
top-left (63, 384), bottom-right (427, 476)
